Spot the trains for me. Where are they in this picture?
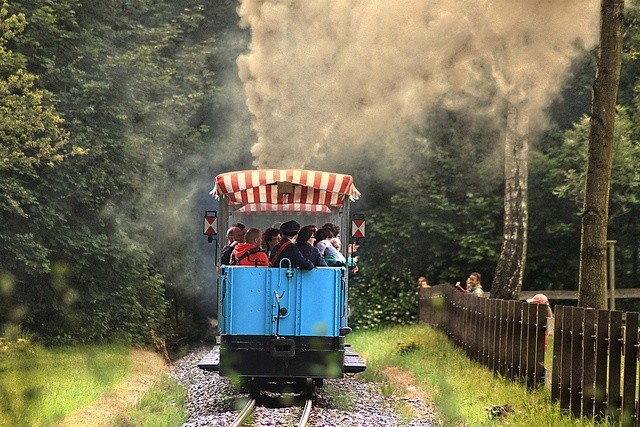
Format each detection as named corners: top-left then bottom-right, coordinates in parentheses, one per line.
top-left (196, 168), bottom-right (366, 399)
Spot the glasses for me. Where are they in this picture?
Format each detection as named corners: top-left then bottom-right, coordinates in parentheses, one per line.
top-left (310, 236), bottom-right (316, 239)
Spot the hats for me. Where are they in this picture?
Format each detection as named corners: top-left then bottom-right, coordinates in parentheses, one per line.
top-left (280, 220), bottom-right (300, 236)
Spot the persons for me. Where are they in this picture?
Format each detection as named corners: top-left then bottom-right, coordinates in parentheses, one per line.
top-left (468, 272), bottom-right (485, 297)
top-left (229, 228), bottom-right (269, 267)
top-left (421, 279), bottom-right (431, 288)
top-left (232, 222), bottom-right (247, 236)
top-left (526, 294), bottom-right (553, 354)
top-left (456, 277), bottom-right (471, 293)
top-left (221, 226), bottom-right (245, 265)
top-left (268, 219), bottom-right (314, 270)
top-left (330, 238), bottom-right (341, 251)
top-left (417, 276), bottom-right (426, 288)
top-left (315, 229), bottom-right (358, 266)
top-left (322, 223), bottom-right (341, 239)
top-left (295, 225), bottom-right (329, 267)
top-left (264, 226), bottom-right (282, 259)
